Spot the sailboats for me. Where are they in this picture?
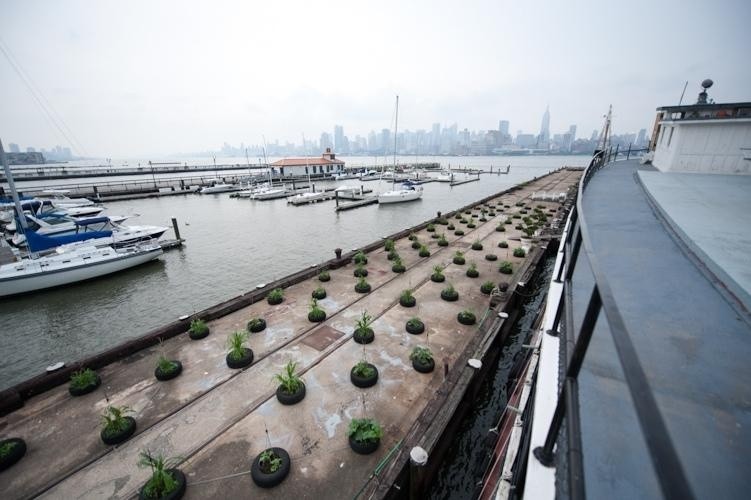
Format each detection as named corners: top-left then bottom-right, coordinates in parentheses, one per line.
top-left (195, 88), bottom-right (471, 216)
top-left (1, 143), bottom-right (175, 301)
